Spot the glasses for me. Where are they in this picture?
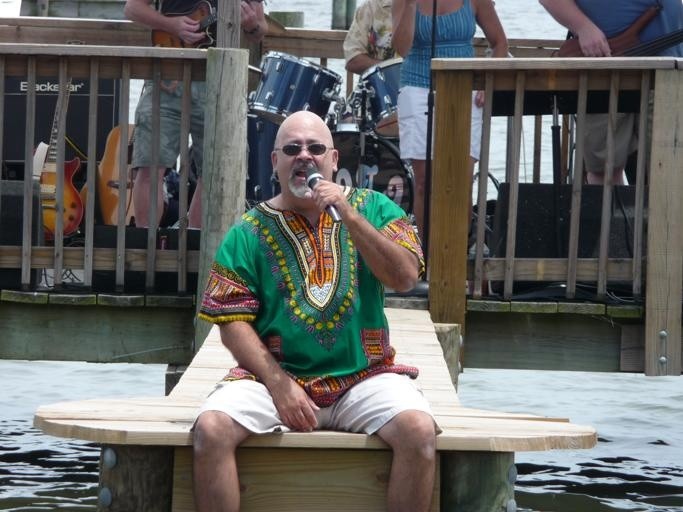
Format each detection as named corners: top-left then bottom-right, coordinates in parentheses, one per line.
top-left (273, 142), bottom-right (335, 155)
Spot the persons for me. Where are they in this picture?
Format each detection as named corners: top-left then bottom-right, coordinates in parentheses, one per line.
top-left (391, 0), bottom-right (507, 247)
top-left (243, 25), bottom-right (260, 35)
top-left (344, 1), bottom-right (402, 77)
top-left (539, 0), bottom-right (683, 185)
top-left (189, 111), bottom-right (442, 512)
top-left (124, 0), bottom-right (268, 228)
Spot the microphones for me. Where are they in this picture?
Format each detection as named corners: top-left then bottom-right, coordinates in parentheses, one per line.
top-left (305, 168), bottom-right (342, 223)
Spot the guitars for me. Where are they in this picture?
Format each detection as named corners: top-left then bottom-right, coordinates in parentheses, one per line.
top-left (151, 1), bottom-right (270, 49)
top-left (32, 41), bottom-right (83, 242)
top-left (559, 6), bottom-right (681, 58)
top-left (79, 81), bottom-right (140, 227)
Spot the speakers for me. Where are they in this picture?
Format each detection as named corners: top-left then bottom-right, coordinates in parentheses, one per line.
top-left (92, 224), bottom-right (201, 296)
top-left (490, 183), bottom-right (649, 301)
top-left (0, 180), bottom-right (44, 290)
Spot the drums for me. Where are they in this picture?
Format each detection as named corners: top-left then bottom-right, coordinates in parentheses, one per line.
top-left (248, 114), bottom-right (280, 204)
top-left (360, 57), bottom-right (403, 138)
top-left (328, 124), bottom-right (414, 217)
top-left (249, 50), bottom-right (341, 124)
